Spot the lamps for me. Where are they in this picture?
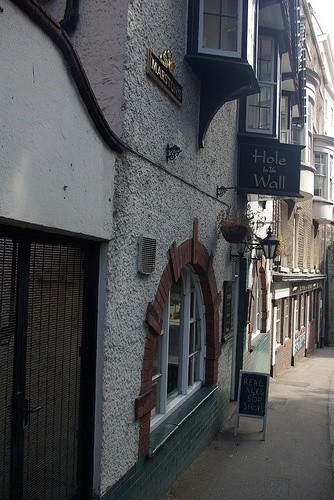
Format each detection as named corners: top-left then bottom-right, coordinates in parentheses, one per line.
top-left (241, 224), bottom-right (281, 259)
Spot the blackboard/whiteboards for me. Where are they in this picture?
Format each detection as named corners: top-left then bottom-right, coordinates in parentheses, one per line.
top-left (237, 142), bottom-right (305, 198)
top-left (236, 369), bottom-right (270, 418)
